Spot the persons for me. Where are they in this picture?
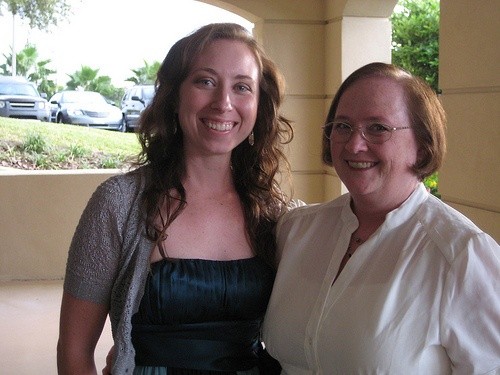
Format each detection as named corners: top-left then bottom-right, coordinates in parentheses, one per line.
top-left (261, 62), bottom-right (500, 375)
top-left (56, 23), bottom-right (294, 375)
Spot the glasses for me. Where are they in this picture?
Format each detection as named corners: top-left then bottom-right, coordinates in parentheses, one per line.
top-left (321, 121), bottom-right (414, 144)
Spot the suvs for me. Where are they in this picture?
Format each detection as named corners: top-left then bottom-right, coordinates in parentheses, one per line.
top-left (121, 84), bottom-right (156, 133)
top-left (0, 75), bottom-right (50, 123)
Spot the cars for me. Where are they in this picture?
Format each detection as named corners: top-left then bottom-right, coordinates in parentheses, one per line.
top-left (49, 90), bottom-right (126, 132)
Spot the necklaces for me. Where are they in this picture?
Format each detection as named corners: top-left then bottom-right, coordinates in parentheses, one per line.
top-left (348, 234), bottom-right (365, 259)
